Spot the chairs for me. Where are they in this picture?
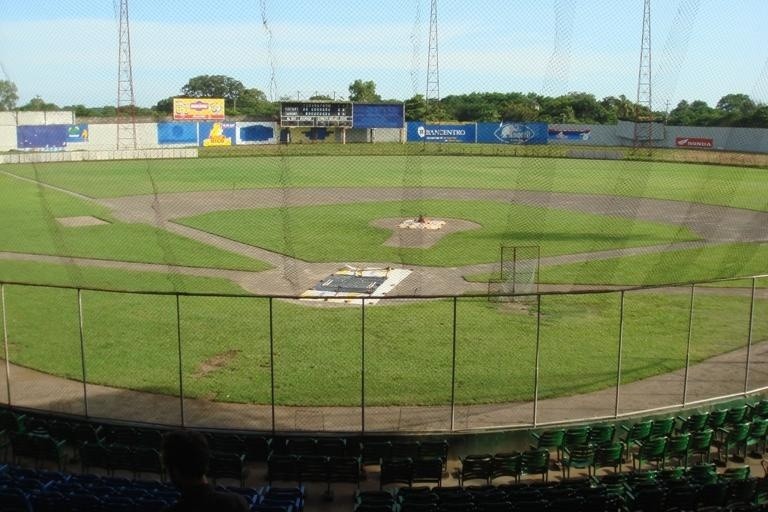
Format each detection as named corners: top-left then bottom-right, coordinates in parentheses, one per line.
top-left (0, 401), bottom-right (768, 512)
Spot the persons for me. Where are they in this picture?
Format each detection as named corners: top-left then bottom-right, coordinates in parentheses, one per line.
top-left (155, 430), bottom-right (248, 511)
